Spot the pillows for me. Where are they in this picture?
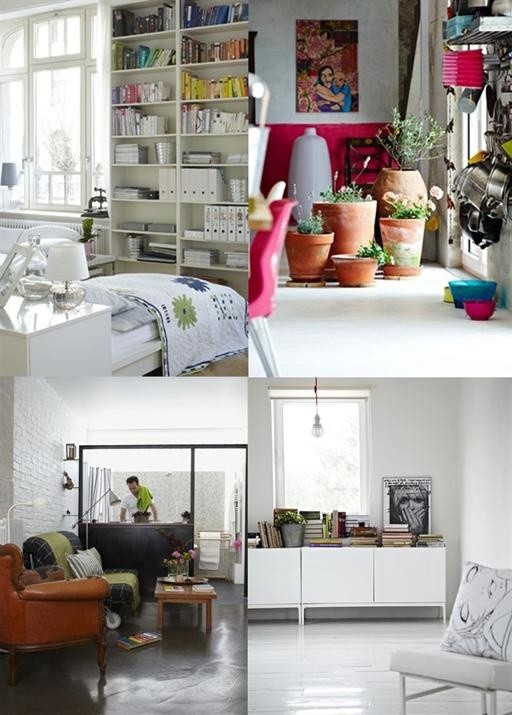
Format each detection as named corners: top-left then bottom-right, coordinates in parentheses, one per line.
top-left (441, 560), bottom-right (512, 662)
top-left (64, 549), bottom-right (105, 580)
top-left (83, 281), bottom-right (135, 314)
top-left (77, 546), bottom-right (102, 564)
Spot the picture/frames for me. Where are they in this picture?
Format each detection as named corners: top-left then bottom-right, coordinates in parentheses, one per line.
top-left (1, 243), bottom-right (37, 312)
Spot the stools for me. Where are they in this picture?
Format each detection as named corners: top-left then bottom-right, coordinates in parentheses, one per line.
top-left (387, 646), bottom-right (512, 715)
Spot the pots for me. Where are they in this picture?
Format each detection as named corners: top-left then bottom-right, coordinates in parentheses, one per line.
top-left (446, 151), bottom-right (512, 249)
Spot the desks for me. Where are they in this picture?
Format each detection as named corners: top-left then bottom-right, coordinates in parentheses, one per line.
top-left (87, 251), bottom-right (116, 278)
top-left (247, 185), bottom-right (274, 232)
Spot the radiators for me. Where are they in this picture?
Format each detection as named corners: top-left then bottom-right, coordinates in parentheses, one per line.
top-left (1, 517), bottom-right (25, 550)
top-left (1, 216), bottom-right (98, 255)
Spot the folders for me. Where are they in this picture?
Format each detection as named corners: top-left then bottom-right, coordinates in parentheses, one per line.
top-left (204, 205), bottom-right (250, 243)
top-left (158, 168), bottom-right (225, 202)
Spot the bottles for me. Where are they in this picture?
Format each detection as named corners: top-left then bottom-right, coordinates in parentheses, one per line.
top-left (457, 71), bottom-right (488, 112)
top-left (139, 46), bottom-right (150, 67)
top-left (24, 234), bottom-right (49, 277)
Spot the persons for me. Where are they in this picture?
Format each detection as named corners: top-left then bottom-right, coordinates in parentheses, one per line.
top-left (315, 65), bottom-right (346, 113)
top-left (118, 475), bottom-right (159, 525)
top-left (390, 485), bottom-right (429, 535)
top-left (313, 71), bottom-right (353, 112)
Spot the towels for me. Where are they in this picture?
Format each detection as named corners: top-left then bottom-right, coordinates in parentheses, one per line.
top-left (137, 485), bottom-right (155, 514)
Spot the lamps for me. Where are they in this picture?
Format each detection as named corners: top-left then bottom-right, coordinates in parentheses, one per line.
top-left (1, 497), bottom-right (52, 545)
top-left (41, 242), bottom-right (89, 308)
top-left (71, 487), bottom-right (121, 550)
top-left (307, 376), bottom-right (323, 438)
top-left (0, 162), bottom-right (19, 211)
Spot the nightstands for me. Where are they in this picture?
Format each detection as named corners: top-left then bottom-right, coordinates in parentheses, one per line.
top-left (1, 295), bottom-right (114, 378)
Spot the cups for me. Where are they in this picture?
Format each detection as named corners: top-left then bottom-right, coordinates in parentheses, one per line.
top-left (176, 576), bottom-right (184, 582)
top-left (229, 177), bottom-right (248, 203)
top-left (154, 142), bottom-right (177, 165)
top-left (124, 238), bottom-right (146, 259)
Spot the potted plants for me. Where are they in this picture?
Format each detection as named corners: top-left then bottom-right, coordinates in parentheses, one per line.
top-left (327, 242), bottom-right (401, 289)
top-left (375, 107), bottom-right (445, 203)
top-left (80, 217), bottom-right (97, 259)
top-left (273, 510), bottom-right (305, 547)
top-left (285, 209), bottom-right (335, 281)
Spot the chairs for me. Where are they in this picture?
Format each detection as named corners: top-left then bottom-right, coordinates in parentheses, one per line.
top-left (339, 134), bottom-right (395, 197)
top-left (249, 198), bottom-right (300, 377)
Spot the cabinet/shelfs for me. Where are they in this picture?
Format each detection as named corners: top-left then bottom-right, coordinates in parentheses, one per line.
top-left (246, 548), bottom-right (302, 629)
top-left (106, 0), bottom-right (248, 300)
top-left (300, 545), bottom-right (446, 629)
top-left (62, 457), bottom-right (82, 489)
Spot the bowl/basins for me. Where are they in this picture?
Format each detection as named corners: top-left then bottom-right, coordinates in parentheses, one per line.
top-left (464, 299), bottom-right (495, 322)
top-left (446, 280), bottom-right (498, 309)
top-left (17, 278), bottom-right (52, 300)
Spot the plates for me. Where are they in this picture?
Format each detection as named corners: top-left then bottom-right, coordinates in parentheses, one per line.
top-left (191, 577), bottom-right (208, 584)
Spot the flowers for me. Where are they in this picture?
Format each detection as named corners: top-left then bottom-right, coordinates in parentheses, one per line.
top-left (310, 153), bottom-right (375, 200)
top-left (232, 540), bottom-right (241, 563)
top-left (164, 545), bottom-right (197, 565)
top-left (381, 184), bottom-right (447, 220)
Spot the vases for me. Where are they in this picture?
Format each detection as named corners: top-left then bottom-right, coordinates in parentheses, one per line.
top-left (377, 216), bottom-right (427, 277)
top-left (311, 203), bottom-right (381, 271)
top-left (177, 564), bottom-right (189, 578)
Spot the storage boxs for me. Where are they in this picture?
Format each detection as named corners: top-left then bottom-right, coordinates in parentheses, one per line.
top-left (442, 15), bottom-right (474, 40)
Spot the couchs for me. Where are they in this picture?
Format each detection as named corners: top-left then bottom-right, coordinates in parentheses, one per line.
top-left (0, 542), bottom-right (118, 686)
top-left (25, 526), bottom-right (139, 623)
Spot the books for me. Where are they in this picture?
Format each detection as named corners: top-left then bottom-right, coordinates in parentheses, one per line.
top-left (115, 629), bottom-right (162, 651)
top-left (191, 584), bottom-right (214, 593)
top-left (163, 585), bottom-right (185, 592)
top-left (110, 0), bottom-right (249, 270)
top-left (256, 508), bottom-right (446, 548)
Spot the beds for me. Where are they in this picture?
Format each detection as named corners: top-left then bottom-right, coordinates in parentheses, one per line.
top-left (150, 572), bottom-right (218, 633)
top-left (84, 262), bottom-right (248, 377)
